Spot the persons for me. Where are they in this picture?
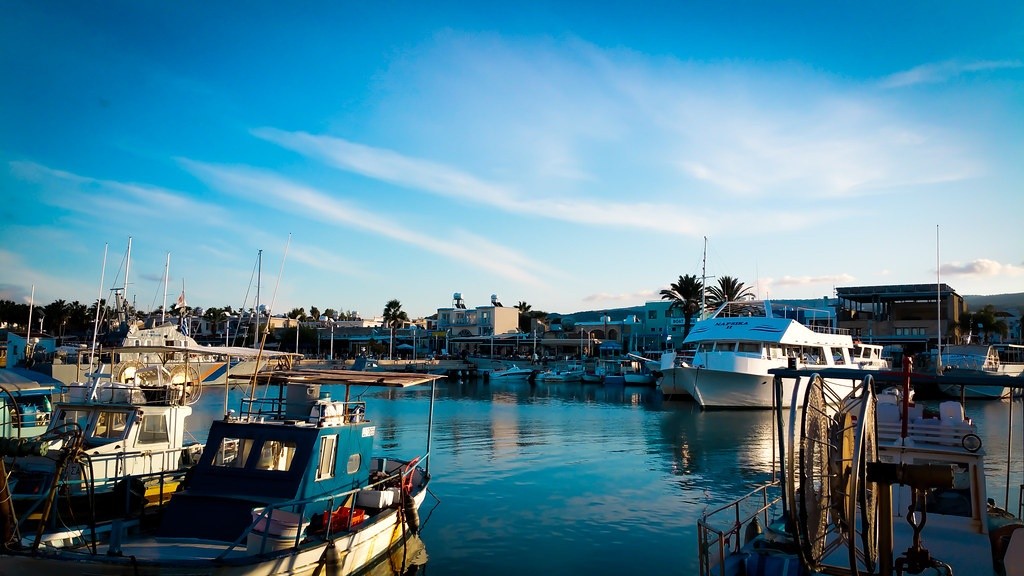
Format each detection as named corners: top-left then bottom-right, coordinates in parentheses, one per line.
top-left (912, 353), bottom-right (931, 372)
top-left (306, 349), bottom-right (549, 364)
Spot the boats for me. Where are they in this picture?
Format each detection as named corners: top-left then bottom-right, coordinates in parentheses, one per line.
top-left (488, 364), bottom-right (532, 380)
top-left (673, 298), bottom-right (888, 411)
top-left (0, 234), bottom-right (239, 549)
top-left (535, 365), bottom-right (605, 381)
top-left (598, 356), bottom-right (658, 386)
top-left (644, 345), bottom-right (693, 398)
top-left (49, 230), bottom-right (449, 576)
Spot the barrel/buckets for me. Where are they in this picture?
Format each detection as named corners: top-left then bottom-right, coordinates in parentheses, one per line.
top-left (247, 530), bottom-right (307, 557)
top-left (252, 508), bottom-right (308, 534)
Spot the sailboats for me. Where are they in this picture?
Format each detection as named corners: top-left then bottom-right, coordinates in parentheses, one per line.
top-left (911, 226), bottom-right (1024, 398)
top-left (36, 235), bottom-right (302, 389)
